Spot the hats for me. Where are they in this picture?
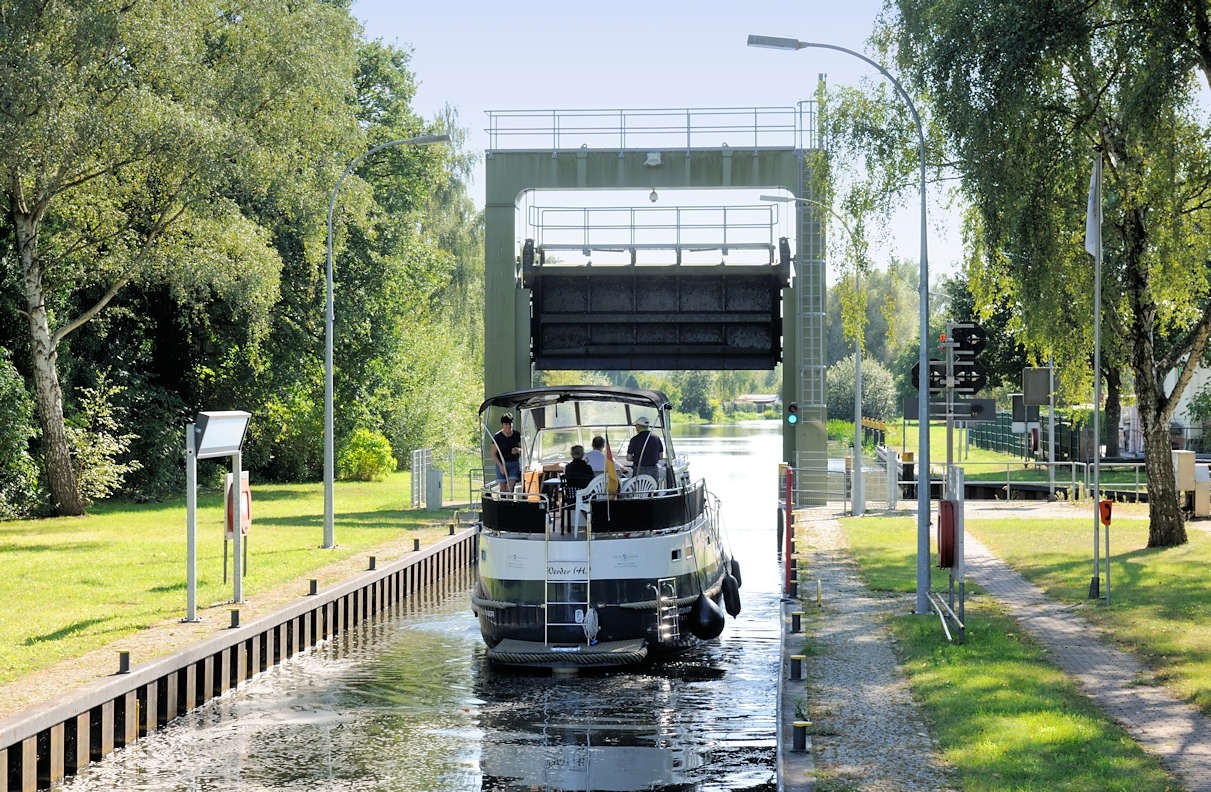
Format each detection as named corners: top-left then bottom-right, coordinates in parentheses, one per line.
top-left (633, 417), bottom-right (650, 426)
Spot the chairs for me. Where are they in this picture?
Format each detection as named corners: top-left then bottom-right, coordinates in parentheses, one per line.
top-left (561, 470), bottom-right (657, 539)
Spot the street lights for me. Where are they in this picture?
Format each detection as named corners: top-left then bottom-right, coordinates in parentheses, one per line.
top-left (759, 192), bottom-right (865, 518)
top-left (742, 31), bottom-right (935, 619)
top-left (323, 133), bottom-right (454, 548)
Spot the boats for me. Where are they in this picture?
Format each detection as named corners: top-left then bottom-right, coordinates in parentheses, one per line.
top-left (466, 386), bottom-right (746, 674)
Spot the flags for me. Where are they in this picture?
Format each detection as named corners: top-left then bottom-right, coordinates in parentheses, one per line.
top-left (605, 433), bottom-right (618, 499)
top-left (1086, 160), bottom-right (1103, 261)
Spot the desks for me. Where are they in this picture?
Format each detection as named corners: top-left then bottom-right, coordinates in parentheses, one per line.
top-left (544, 478), bottom-right (566, 532)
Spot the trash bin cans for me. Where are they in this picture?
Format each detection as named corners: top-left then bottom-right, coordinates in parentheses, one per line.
top-left (1171, 449), bottom-right (1195, 492)
top-left (426, 470), bottom-right (444, 510)
top-left (1196, 463), bottom-right (1210, 518)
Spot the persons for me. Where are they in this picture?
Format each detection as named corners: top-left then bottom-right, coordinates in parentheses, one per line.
top-left (627, 417), bottom-right (663, 497)
top-left (582, 436), bottom-right (625, 471)
top-left (565, 445), bottom-right (596, 520)
top-left (492, 412), bottom-right (521, 499)
